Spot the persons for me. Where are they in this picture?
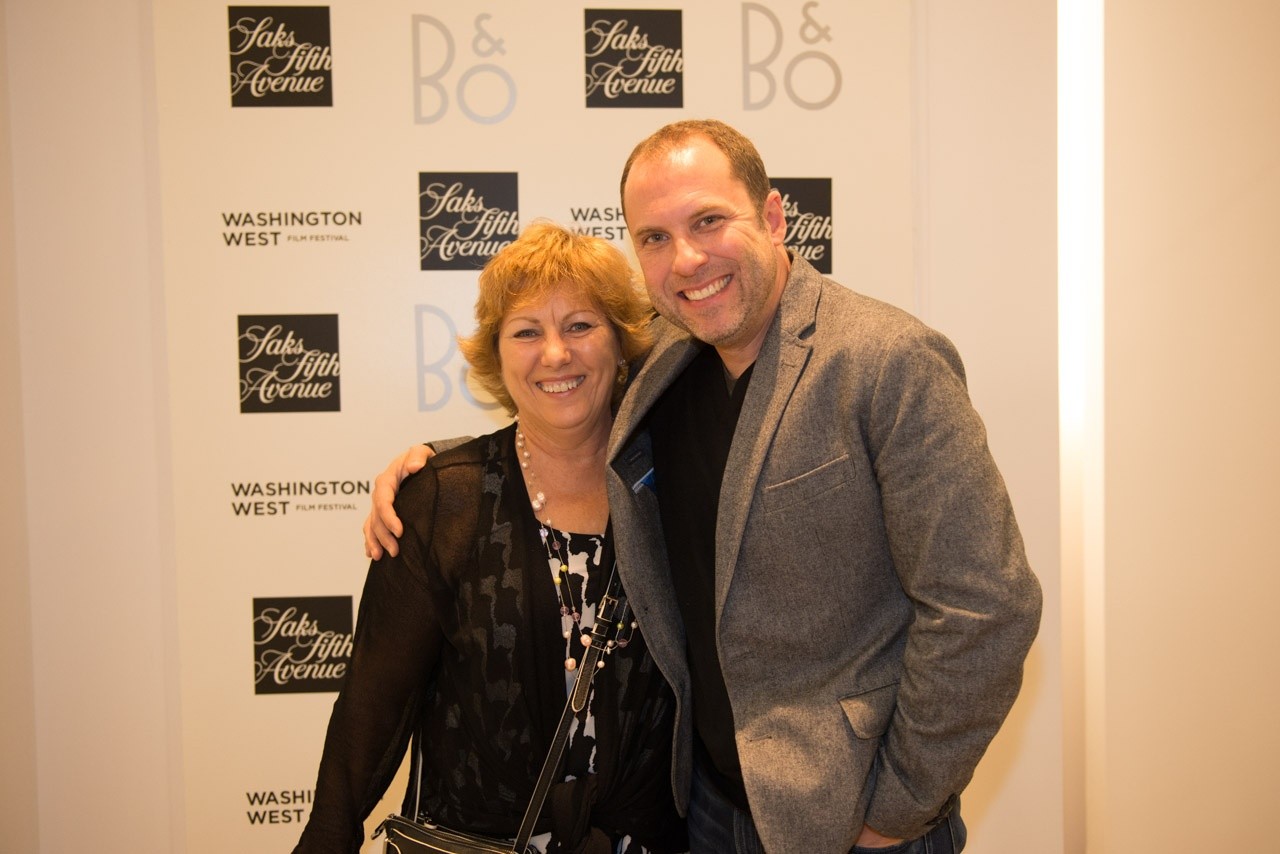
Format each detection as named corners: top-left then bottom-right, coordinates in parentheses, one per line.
top-left (287, 219), bottom-right (686, 854)
top-left (363, 121), bottom-right (1041, 854)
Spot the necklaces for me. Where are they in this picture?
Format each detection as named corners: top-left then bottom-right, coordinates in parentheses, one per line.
top-left (516, 425), bottom-right (638, 682)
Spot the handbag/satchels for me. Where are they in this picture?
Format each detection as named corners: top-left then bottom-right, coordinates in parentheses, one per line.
top-left (378, 815), bottom-right (537, 854)
